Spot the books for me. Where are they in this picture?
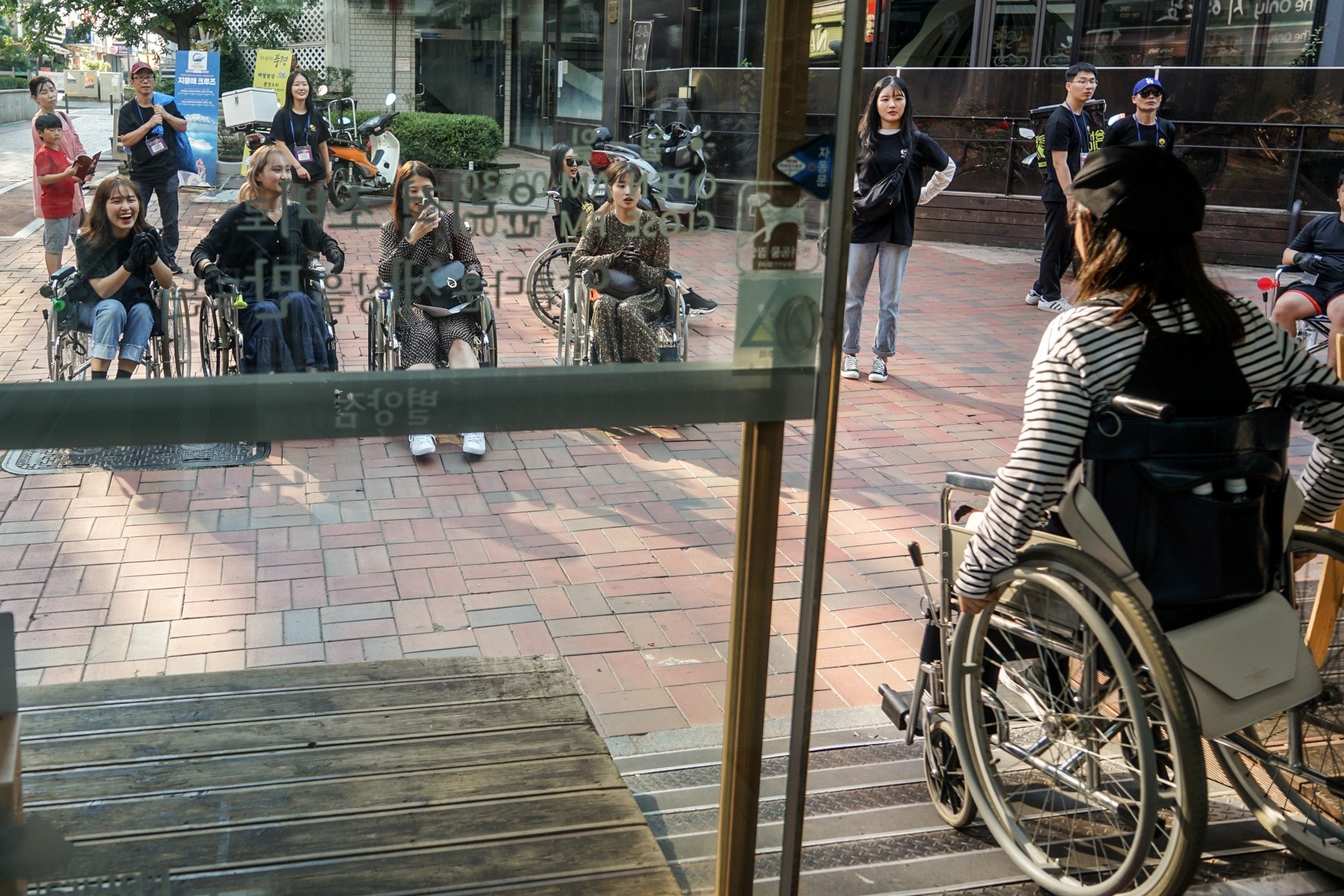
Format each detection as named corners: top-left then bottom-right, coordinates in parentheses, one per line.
top-left (70, 149), bottom-right (102, 183)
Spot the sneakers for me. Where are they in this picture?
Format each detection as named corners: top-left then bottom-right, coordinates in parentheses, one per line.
top-left (167, 260), bottom-right (182, 273)
top-left (1025, 290), bottom-right (1042, 305)
top-left (463, 432), bottom-right (486, 455)
top-left (840, 354), bottom-right (859, 379)
top-left (679, 288), bottom-right (718, 316)
top-left (868, 356), bottom-right (888, 382)
top-left (1037, 295), bottom-right (1073, 314)
top-left (408, 433), bottom-right (437, 456)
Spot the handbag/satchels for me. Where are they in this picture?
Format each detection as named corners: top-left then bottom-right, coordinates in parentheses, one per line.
top-left (595, 266), bottom-right (641, 299)
top-left (851, 164), bottom-right (905, 238)
top-left (1162, 589), bottom-right (1321, 739)
top-left (417, 259), bottom-right (478, 310)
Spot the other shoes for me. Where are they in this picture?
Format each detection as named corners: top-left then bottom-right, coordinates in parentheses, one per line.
top-left (40, 276), bottom-right (71, 298)
top-left (999, 659), bottom-right (1070, 722)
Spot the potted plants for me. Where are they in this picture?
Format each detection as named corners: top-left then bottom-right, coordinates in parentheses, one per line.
top-left (381, 113), bottom-right (500, 201)
top-left (301, 66), bottom-right (358, 110)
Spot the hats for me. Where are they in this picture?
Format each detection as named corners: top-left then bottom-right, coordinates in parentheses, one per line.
top-left (1063, 140), bottom-right (1205, 239)
top-left (130, 61), bottom-right (154, 79)
top-left (1133, 77), bottom-right (1164, 96)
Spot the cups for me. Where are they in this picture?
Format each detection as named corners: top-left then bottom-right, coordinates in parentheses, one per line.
top-left (469, 160), bottom-right (474, 170)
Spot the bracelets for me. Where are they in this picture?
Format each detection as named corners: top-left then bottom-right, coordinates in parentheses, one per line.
top-left (295, 166), bottom-right (303, 170)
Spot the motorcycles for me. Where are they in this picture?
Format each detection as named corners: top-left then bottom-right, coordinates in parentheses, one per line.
top-left (1018, 99), bottom-right (1126, 278)
top-left (221, 85), bottom-right (332, 171)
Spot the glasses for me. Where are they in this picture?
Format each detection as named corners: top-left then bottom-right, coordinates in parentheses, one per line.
top-left (565, 156), bottom-right (583, 167)
top-left (1070, 78), bottom-right (1099, 87)
top-left (133, 76), bottom-right (154, 81)
top-left (1139, 86), bottom-right (1163, 98)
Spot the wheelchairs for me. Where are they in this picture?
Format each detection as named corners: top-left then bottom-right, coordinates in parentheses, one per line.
top-left (197, 218), bottom-right (343, 377)
top-left (367, 278), bottom-right (498, 371)
top-left (39, 228), bottom-right (197, 381)
top-left (879, 381), bottom-right (1344, 896)
top-left (1263, 263), bottom-right (1331, 356)
top-left (525, 188), bottom-right (690, 366)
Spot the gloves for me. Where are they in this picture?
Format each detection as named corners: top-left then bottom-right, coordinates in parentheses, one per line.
top-left (203, 263), bottom-right (231, 299)
top-left (460, 271), bottom-right (481, 290)
top-left (327, 248), bottom-right (345, 275)
top-left (123, 227), bottom-right (159, 274)
top-left (607, 242), bottom-right (641, 271)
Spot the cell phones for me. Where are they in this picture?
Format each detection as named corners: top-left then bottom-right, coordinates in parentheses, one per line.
top-left (423, 197), bottom-right (439, 228)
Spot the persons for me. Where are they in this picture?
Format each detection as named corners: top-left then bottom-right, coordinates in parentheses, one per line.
top-left (268, 70), bottom-right (333, 271)
top-left (375, 161), bottom-right (487, 456)
top-left (28, 77), bottom-right (96, 275)
top-left (186, 145), bottom-right (345, 375)
top-left (116, 62), bottom-right (188, 274)
top-left (1269, 165), bottom-right (1344, 383)
top-left (57, 176), bottom-right (172, 379)
top-left (1020, 61), bottom-right (1099, 315)
top-left (1099, 77), bottom-right (1176, 153)
top-left (836, 74), bottom-right (956, 383)
top-left (566, 160), bottom-right (670, 365)
top-left (961, 142), bottom-right (1344, 693)
top-left (548, 143), bottom-right (718, 317)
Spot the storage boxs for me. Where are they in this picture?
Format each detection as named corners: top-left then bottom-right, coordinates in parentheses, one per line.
top-left (221, 87), bottom-right (279, 127)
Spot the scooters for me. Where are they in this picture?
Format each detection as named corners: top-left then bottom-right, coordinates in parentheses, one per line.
top-left (326, 92), bottom-right (401, 211)
top-left (586, 97), bottom-right (708, 229)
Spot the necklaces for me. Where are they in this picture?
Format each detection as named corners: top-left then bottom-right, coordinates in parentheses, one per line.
top-left (252, 197), bottom-right (282, 213)
